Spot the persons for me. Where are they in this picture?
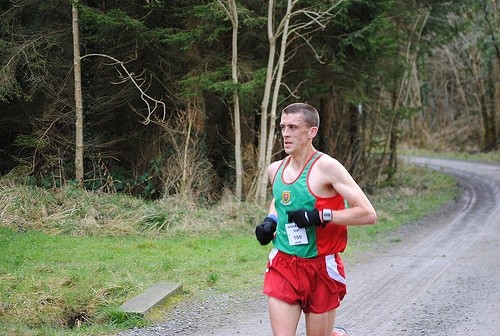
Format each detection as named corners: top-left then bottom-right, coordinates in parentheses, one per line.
top-left (255, 102), bottom-right (377, 336)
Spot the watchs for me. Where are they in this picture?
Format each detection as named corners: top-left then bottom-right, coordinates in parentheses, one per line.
top-left (321, 208), bottom-right (332, 228)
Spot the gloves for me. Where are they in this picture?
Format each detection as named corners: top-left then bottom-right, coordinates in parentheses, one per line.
top-left (255, 217), bottom-right (277, 246)
top-left (286, 208), bottom-right (322, 228)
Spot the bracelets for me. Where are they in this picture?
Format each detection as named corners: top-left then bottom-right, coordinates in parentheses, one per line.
top-left (264, 214), bottom-right (277, 223)
